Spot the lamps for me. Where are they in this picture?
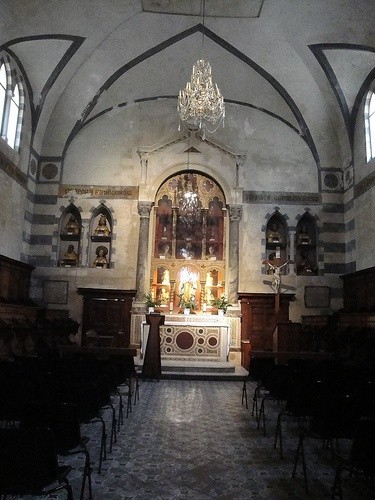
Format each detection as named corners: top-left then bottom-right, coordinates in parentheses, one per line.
top-left (176, 0), bottom-right (226, 141)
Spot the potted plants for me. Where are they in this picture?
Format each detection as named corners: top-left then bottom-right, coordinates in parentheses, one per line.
top-left (211, 297), bottom-right (233, 316)
top-left (143, 293), bottom-right (155, 313)
top-left (182, 302), bottom-right (194, 315)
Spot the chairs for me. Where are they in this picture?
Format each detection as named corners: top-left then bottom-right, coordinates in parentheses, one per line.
top-left (241, 356), bottom-right (375, 500)
top-left (0, 354), bottom-right (141, 499)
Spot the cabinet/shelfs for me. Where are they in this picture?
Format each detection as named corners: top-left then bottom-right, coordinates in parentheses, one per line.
top-left (59, 233), bottom-right (79, 266)
top-left (91, 235), bottom-right (111, 268)
top-left (155, 210), bottom-right (224, 261)
top-left (267, 241), bottom-right (287, 276)
top-left (294, 243), bottom-right (316, 276)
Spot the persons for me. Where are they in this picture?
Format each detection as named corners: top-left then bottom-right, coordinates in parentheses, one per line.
top-left (60, 195), bottom-right (316, 313)
